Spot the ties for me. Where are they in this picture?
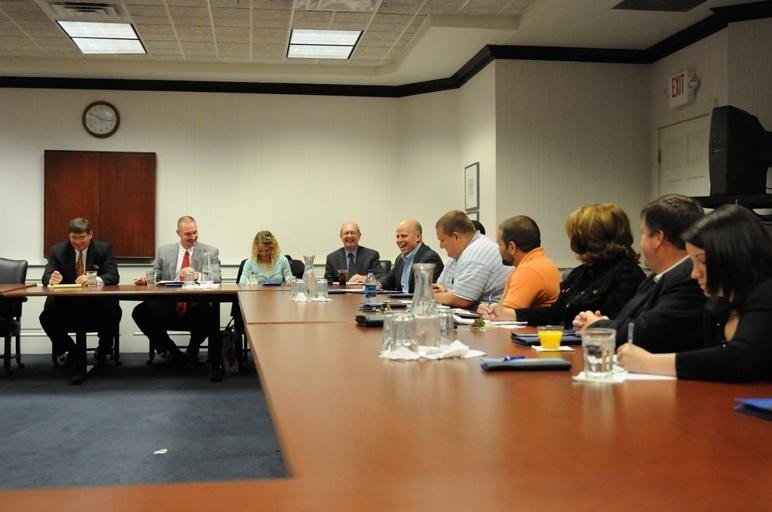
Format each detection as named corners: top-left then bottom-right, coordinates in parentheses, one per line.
top-left (179, 249), bottom-right (192, 282)
top-left (73, 248), bottom-right (86, 283)
top-left (345, 251), bottom-right (357, 280)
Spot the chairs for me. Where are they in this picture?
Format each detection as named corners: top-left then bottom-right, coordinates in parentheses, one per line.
top-left (233, 258), bottom-right (304, 361)
top-left (0, 258), bottom-right (30, 370)
top-left (379, 260), bottom-right (391, 271)
top-left (148, 259), bottom-right (222, 363)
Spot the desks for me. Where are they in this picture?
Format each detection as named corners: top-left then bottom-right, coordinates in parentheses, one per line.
top-left (0, 282), bottom-right (384, 296)
top-left (238, 292), bottom-right (771, 510)
top-left (3, 475), bottom-right (296, 511)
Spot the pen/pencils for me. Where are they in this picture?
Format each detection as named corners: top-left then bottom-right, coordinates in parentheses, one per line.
top-left (488, 290), bottom-right (493, 304)
top-left (503, 356), bottom-right (526, 362)
top-left (628, 322), bottom-right (635, 343)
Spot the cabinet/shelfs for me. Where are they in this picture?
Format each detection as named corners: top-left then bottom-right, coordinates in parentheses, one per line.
top-left (43, 148), bottom-right (157, 260)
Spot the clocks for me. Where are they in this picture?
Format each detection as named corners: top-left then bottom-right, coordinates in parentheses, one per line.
top-left (83, 101), bottom-right (120, 138)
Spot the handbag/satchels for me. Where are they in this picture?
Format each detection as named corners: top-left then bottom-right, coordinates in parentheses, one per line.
top-left (215, 315), bottom-right (248, 378)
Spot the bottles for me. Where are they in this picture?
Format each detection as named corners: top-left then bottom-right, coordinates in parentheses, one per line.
top-left (202, 252), bottom-right (214, 287)
top-left (364, 270), bottom-right (376, 302)
top-left (302, 256), bottom-right (318, 298)
top-left (411, 263), bottom-right (440, 354)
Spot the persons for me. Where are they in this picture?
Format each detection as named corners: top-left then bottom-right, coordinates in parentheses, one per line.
top-left (368, 220), bottom-right (442, 287)
top-left (39, 219), bottom-right (124, 383)
top-left (238, 231), bottom-right (294, 288)
top-left (132, 215), bottom-right (220, 370)
top-left (326, 223), bottom-right (383, 287)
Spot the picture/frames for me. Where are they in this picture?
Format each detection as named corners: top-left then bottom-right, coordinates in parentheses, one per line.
top-left (464, 161), bottom-right (480, 211)
top-left (465, 212), bottom-right (479, 222)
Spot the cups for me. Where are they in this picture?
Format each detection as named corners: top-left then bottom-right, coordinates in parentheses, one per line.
top-left (250, 269), bottom-right (258, 286)
top-left (338, 270), bottom-right (347, 285)
top-left (86, 271), bottom-right (97, 287)
top-left (290, 279), bottom-right (328, 299)
top-left (383, 307), bottom-right (456, 355)
top-left (184, 272), bottom-right (196, 289)
top-left (146, 270), bottom-right (157, 287)
top-left (538, 325), bottom-right (565, 350)
top-left (581, 328), bottom-right (616, 379)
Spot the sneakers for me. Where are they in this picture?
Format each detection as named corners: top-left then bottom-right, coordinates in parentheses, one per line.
top-left (93, 344), bottom-right (126, 372)
top-left (56, 343), bottom-right (90, 371)
top-left (162, 341), bottom-right (206, 372)
